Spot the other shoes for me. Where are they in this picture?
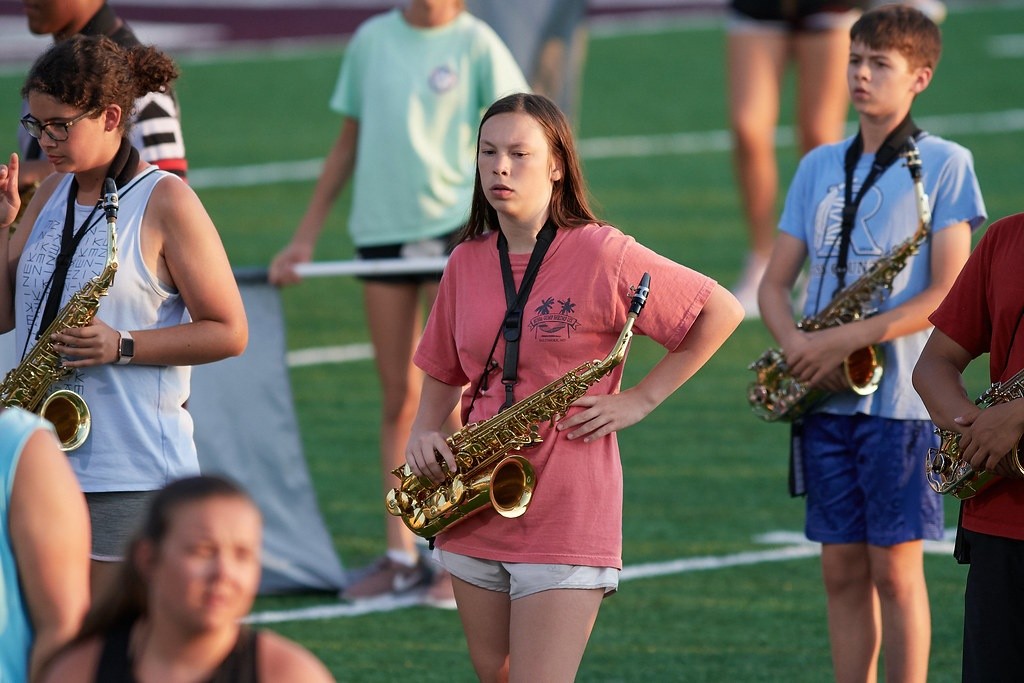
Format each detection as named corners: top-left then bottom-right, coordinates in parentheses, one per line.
top-left (735, 262), bottom-right (767, 318)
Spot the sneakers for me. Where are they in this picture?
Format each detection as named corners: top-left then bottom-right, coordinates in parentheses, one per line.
top-left (342, 555), bottom-right (435, 604)
top-left (425, 568), bottom-right (456, 609)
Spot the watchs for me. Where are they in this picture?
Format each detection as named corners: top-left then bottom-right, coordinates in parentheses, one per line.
top-left (113, 330), bottom-right (135, 365)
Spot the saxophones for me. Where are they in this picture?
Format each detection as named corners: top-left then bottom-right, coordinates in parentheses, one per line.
top-left (925, 378), bottom-right (1024, 496)
top-left (748, 134), bottom-right (931, 420)
top-left (0, 178), bottom-right (121, 450)
top-left (387, 272), bottom-right (650, 549)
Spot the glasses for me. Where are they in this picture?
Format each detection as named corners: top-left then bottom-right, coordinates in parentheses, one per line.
top-left (22, 105), bottom-right (99, 141)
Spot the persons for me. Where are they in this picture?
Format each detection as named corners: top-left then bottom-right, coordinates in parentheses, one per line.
top-left (752, 4), bottom-right (990, 683)
top-left (910, 211), bottom-right (1024, 683)
top-left (31, 473), bottom-right (337, 683)
top-left (0, 34), bottom-right (249, 607)
top-left (269, 0), bottom-right (534, 610)
top-left (405, 93), bottom-right (746, 683)
top-left (0, 0), bottom-right (189, 182)
top-left (0, 400), bottom-right (94, 683)
top-left (714, 1), bottom-right (873, 319)
